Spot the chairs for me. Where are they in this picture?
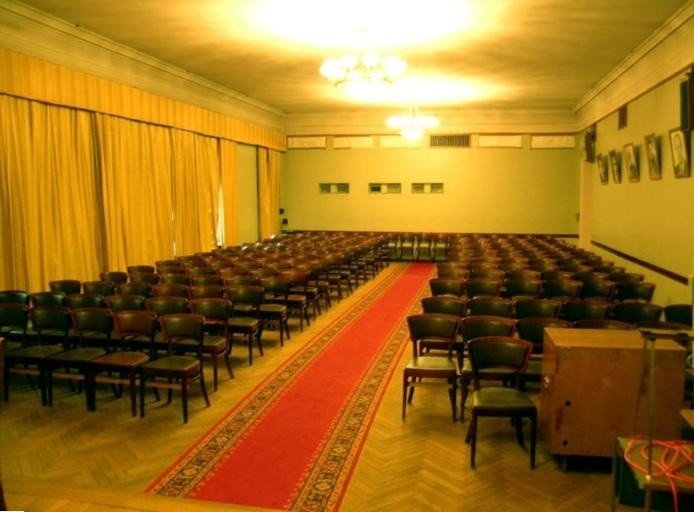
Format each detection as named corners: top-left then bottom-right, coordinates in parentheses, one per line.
top-left (0, 229), bottom-right (391, 423)
top-left (392, 231), bottom-right (694, 470)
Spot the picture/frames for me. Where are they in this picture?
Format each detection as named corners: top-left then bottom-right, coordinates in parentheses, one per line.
top-left (594, 153), bottom-right (607, 186)
top-left (607, 147), bottom-right (621, 184)
top-left (643, 131), bottom-right (661, 182)
top-left (667, 126), bottom-right (691, 179)
top-left (621, 140), bottom-right (640, 184)
